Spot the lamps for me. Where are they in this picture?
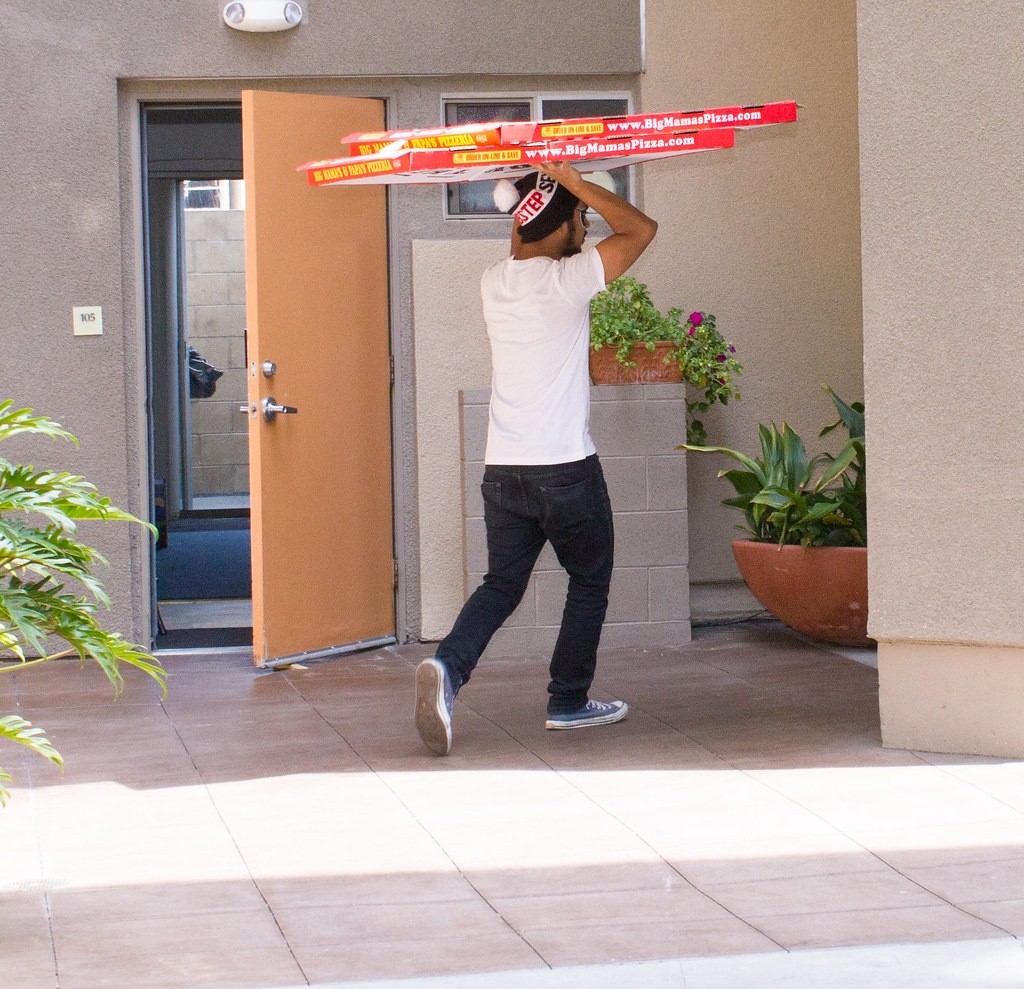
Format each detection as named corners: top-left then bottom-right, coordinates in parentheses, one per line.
top-left (223, 0), bottom-right (303, 32)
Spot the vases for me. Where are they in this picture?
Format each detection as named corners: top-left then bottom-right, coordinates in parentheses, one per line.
top-left (589, 341), bottom-right (686, 386)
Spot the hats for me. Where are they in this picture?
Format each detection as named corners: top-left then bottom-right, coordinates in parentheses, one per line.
top-left (493, 171), bottom-right (579, 243)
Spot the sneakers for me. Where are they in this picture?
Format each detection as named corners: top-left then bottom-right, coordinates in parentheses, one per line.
top-left (546, 700), bottom-right (628, 729)
top-left (416, 657), bottom-right (455, 755)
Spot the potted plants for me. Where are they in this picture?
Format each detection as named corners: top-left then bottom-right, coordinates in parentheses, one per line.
top-left (675, 383), bottom-right (876, 647)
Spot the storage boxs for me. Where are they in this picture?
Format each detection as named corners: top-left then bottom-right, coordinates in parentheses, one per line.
top-left (296, 102), bottom-right (803, 186)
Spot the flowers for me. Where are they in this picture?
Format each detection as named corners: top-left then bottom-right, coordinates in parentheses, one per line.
top-left (588, 276), bottom-right (745, 447)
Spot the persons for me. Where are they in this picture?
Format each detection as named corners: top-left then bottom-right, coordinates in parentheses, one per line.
top-left (416, 159), bottom-right (658, 756)
